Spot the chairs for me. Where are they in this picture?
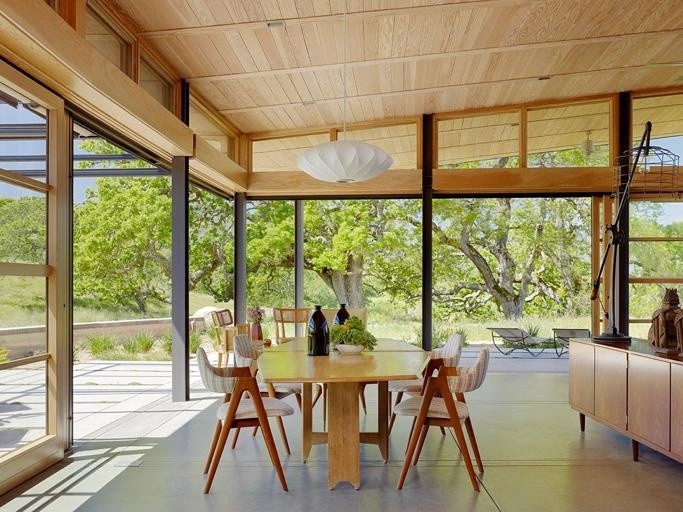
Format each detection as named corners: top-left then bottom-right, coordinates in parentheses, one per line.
top-left (394, 346), bottom-right (492, 491)
top-left (552, 328), bottom-right (592, 357)
top-left (487, 328), bottom-right (549, 357)
top-left (389, 333), bottom-right (477, 456)
top-left (321, 308), bottom-right (367, 328)
top-left (231, 333), bottom-right (293, 454)
top-left (210, 309), bottom-right (236, 369)
top-left (274, 308), bottom-right (313, 344)
top-left (196, 347), bottom-right (293, 494)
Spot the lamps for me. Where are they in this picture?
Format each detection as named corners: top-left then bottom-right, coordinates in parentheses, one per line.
top-left (589, 121), bottom-right (681, 344)
top-left (297, 1), bottom-right (392, 183)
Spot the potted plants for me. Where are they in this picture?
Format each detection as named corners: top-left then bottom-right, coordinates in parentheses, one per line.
top-left (329, 315), bottom-right (377, 355)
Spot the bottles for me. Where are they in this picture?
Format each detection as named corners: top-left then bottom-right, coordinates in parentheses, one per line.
top-left (306, 303), bottom-right (331, 357)
top-left (333, 302), bottom-right (353, 330)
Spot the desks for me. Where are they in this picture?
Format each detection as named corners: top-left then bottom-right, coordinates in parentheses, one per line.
top-left (563, 345), bottom-right (628, 436)
top-left (264, 338), bottom-right (426, 460)
top-left (257, 338), bottom-right (417, 490)
top-left (627, 348), bottom-right (683, 465)
